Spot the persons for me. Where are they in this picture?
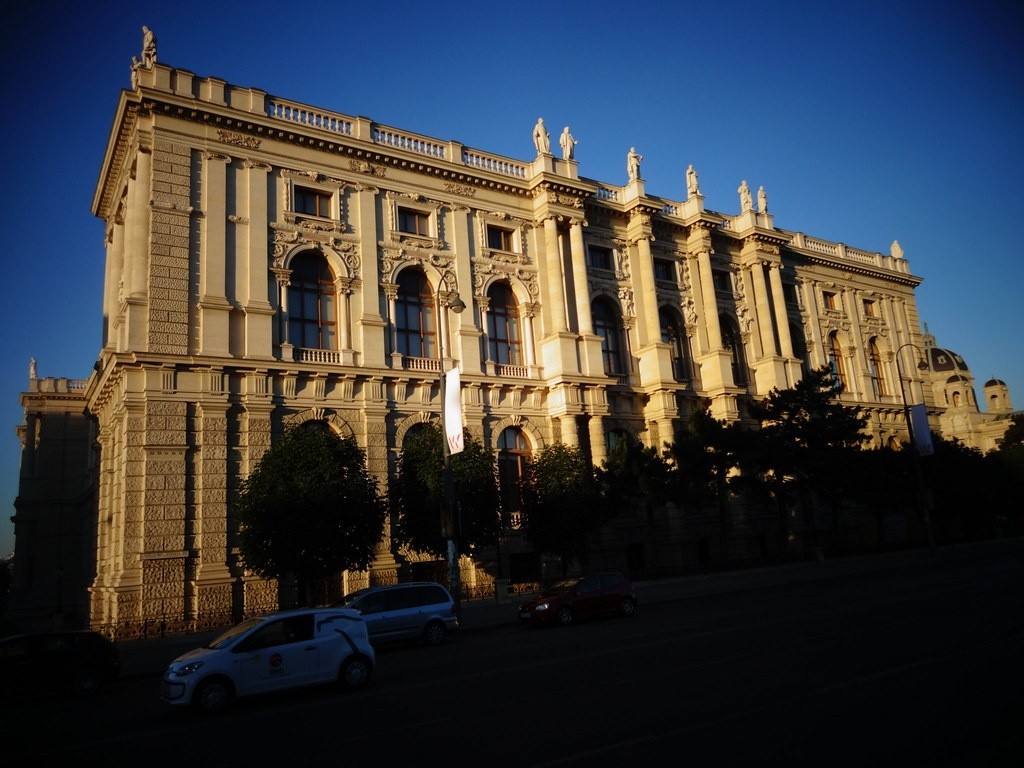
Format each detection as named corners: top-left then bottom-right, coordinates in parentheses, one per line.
top-left (889, 239), bottom-right (905, 259)
top-left (535, 115), bottom-right (769, 212)
top-left (140, 25), bottom-right (156, 67)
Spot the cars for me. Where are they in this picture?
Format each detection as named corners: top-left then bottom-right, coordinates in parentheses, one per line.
top-left (332, 581), bottom-right (460, 645)
top-left (519, 573), bottom-right (635, 622)
top-left (167, 608), bottom-right (374, 717)
